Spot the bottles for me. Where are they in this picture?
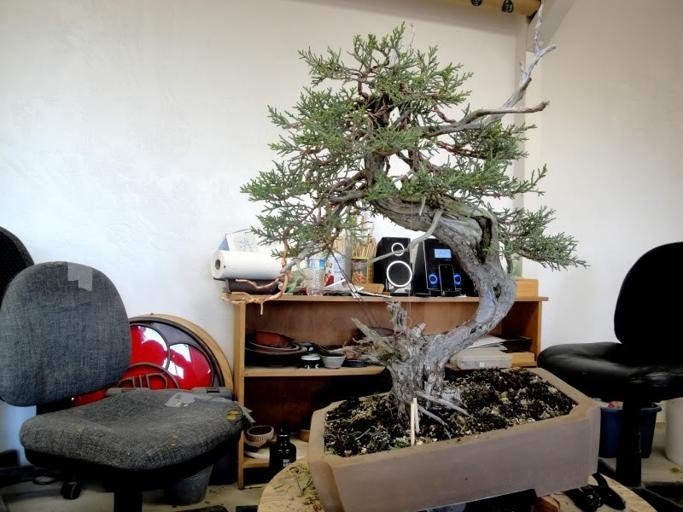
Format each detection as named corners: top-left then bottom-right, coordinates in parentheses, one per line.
top-left (267, 434), bottom-right (296, 480)
top-left (304, 245), bottom-right (325, 296)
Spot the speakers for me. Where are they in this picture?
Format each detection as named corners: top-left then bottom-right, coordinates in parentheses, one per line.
top-left (373, 237), bottom-right (413, 297)
top-left (422, 237), bottom-right (469, 297)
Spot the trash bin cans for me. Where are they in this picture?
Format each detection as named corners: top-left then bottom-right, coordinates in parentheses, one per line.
top-left (600, 396), bottom-right (662, 459)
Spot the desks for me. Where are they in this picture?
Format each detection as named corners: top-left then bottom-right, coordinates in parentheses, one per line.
top-left (256, 456), bottom-right (658, 512)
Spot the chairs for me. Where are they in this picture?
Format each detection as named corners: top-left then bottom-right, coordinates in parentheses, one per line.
top-left (0, 260), bottom-right (243, 512)
top-left (539, 241), bottom-right (683, 512)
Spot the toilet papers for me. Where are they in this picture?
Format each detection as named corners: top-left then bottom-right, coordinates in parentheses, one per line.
top-left (212, 250), bottom-right (306, 280)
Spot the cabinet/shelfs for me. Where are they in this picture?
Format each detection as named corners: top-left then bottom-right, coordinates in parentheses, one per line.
top-left (228, 294), bottom-right (550, 490)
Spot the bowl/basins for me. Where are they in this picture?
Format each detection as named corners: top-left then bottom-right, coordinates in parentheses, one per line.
top-left (246, 425), bottom-right (274, 441)
top-left (299, 424), bottom-right (310, 440)
top-left (244, 327), bottom-right (393, 368)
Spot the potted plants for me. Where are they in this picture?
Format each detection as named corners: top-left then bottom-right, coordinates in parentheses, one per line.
top-left (239, 15), bottom-right (601, 512)
